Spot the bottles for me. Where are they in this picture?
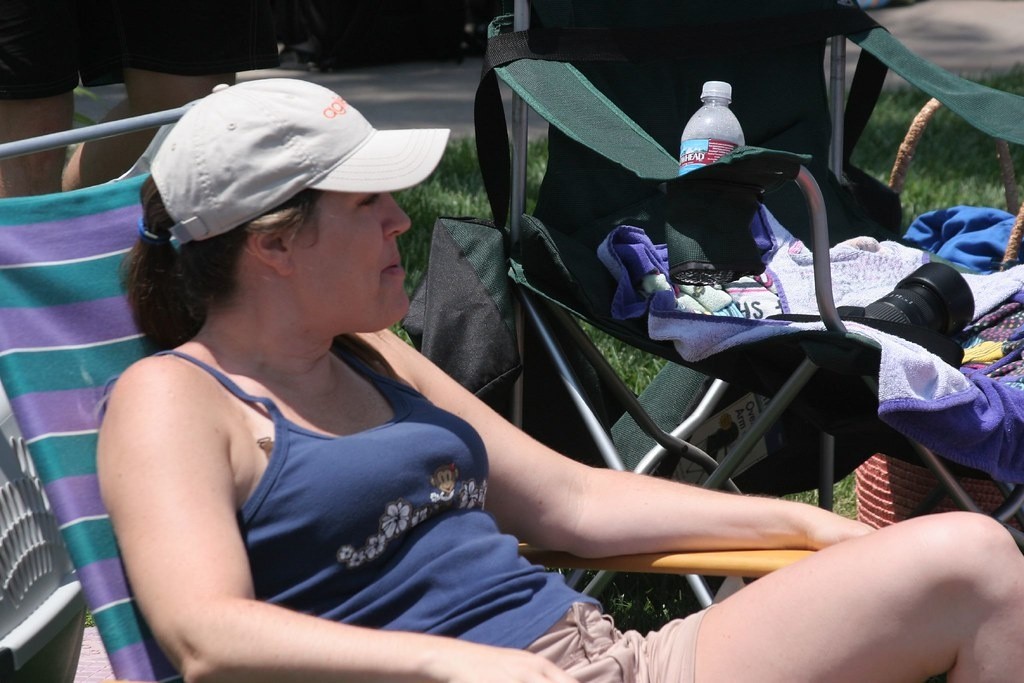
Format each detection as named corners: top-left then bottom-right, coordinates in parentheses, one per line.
top-left (669, 81), bottom-right (745, 286)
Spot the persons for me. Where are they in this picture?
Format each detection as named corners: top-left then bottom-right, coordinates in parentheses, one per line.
top-left (1, 0), bottom-right (317, 198)
top-left (95, 77), bottom-right (1024, 683)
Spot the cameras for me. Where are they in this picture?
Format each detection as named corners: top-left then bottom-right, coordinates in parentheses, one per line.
top-left (835, 262), bottom-right (976, 338)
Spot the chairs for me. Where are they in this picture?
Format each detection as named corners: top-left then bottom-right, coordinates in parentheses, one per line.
top-left (0, 98), bottom-right (817, 682)
top-left (488, 0), bottom-right (1023, 619)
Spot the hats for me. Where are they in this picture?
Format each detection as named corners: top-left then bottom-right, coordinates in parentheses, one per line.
top-left (137, 78), bottom-right (451, 246)
